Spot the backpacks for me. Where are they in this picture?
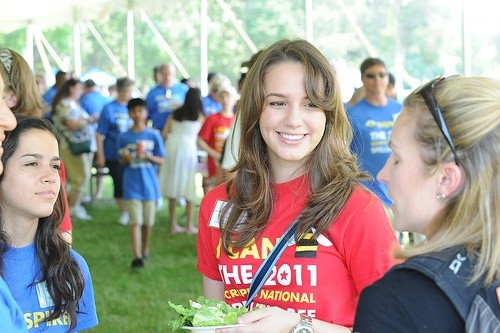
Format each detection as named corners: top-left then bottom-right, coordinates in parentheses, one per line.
top-left (385, 254), bottom-right (500, 333)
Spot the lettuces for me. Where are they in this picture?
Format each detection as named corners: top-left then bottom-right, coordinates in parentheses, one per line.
top-left (164, 295), bottom-right (265, 332)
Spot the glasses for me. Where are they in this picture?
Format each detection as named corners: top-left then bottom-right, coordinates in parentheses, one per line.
top-left (166, 89), bottom-right (172, 99)
top-left (414, 75), bottom-right (460, 166)
top-left (363, 72), bottom-right (389, 79)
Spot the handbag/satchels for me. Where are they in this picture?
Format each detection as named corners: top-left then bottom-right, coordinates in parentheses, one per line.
top-left (69, 140), bottom-right (91, 154)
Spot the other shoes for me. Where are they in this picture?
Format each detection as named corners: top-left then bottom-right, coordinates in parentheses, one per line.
top-left (119, 211), bottom-right (130, 225)
top-left (187, 227), bottom-right (198, 234)
top-left (170, 226), bottom-right (187, 233)
top-left (69, 205), bottom-right (91, 220)
top-left (132, 257), bottom-right (143, 268)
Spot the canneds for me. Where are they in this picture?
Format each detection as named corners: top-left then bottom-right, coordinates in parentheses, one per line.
top-left (136, 141), bottom-right (145, 158)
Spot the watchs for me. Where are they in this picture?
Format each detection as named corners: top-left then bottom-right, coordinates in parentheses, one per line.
top-left (290, 313), bottom-right (314, 333)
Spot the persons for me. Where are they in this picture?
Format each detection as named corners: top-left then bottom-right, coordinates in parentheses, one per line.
top-left (1, 48), bottom-right (410, 270)
top-left (197, 38), bottom-right (413, 333)
top-left (353, 75), bottom-right (500, 333)
top-left (0, 119), bottom-right (99, 333)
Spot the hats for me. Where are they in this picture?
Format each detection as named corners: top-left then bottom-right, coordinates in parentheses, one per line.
top-left (218, 85), bottom-right (237, 101)
